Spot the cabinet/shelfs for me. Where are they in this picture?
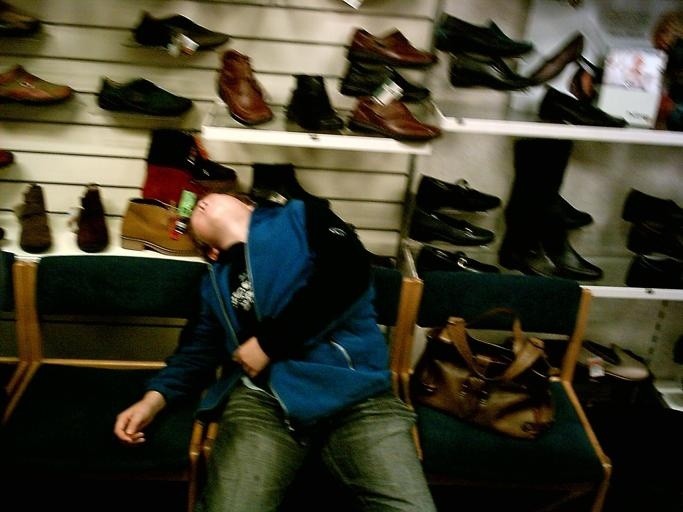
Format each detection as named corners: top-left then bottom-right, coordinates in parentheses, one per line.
top-left (1, 123), bottom-right (433, 265)
top-left (401, 1), bottom-right (683, 413)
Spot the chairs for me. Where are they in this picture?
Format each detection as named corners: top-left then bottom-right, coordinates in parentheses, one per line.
top-left (1, 255), bottom-right (203, 511)
top-left (1, 253), bottom-right (30, 412)
top-left (203, 253), bottom-right (411, 486)
top-left (399, 274), bottom-right (611, 512)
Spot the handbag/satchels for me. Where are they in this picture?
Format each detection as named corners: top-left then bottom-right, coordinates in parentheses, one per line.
top-left (410, 317), bottom-right (558, 441)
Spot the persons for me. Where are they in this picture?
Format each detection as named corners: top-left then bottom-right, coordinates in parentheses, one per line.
top-left (114, 192), bottom-right (439, 512)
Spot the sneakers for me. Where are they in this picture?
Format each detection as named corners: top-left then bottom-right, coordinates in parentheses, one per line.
top-left (577, 340), bottom-right (650, 381)
